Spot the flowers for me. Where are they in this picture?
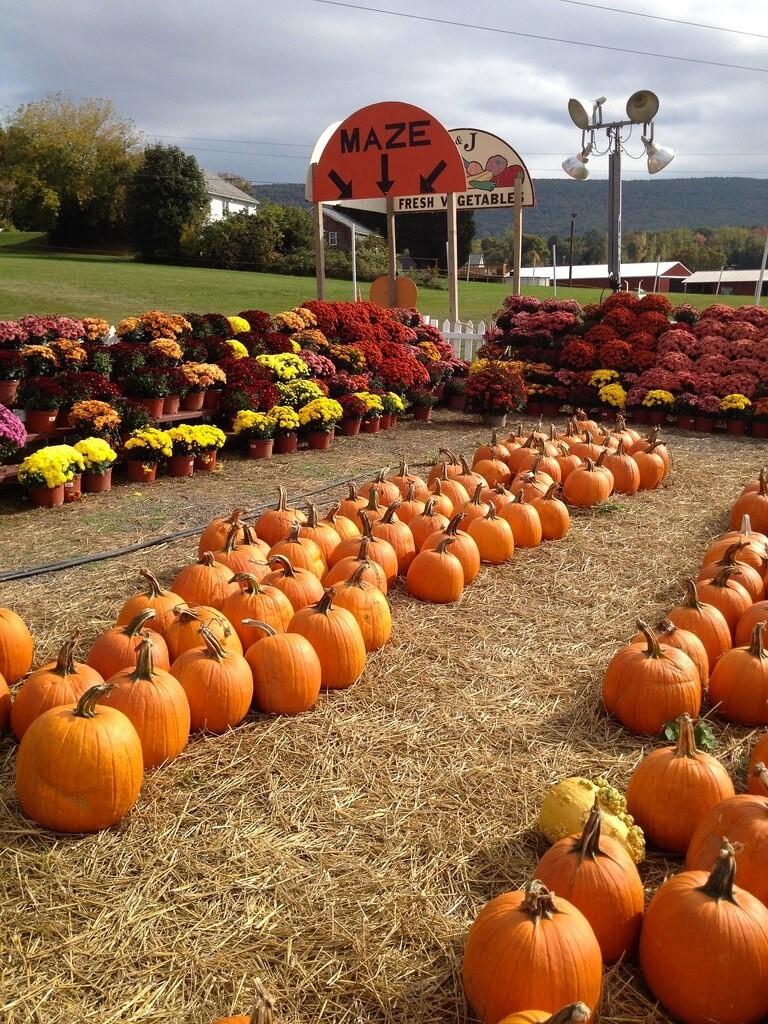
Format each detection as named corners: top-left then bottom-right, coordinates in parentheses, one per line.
top-left (1, 301), bottom-right (470, 490)
top-left (470, 293), bottom-right (768, 421)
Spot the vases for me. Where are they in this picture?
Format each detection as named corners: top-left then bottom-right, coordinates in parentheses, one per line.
top-left (485, 399), bottom-right (768, 439)
top-left (0, 379), bottom-right (468, 508)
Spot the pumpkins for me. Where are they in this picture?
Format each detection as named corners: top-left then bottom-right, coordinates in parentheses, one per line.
top-left (0, 409), bottom-right (768, 1024)
